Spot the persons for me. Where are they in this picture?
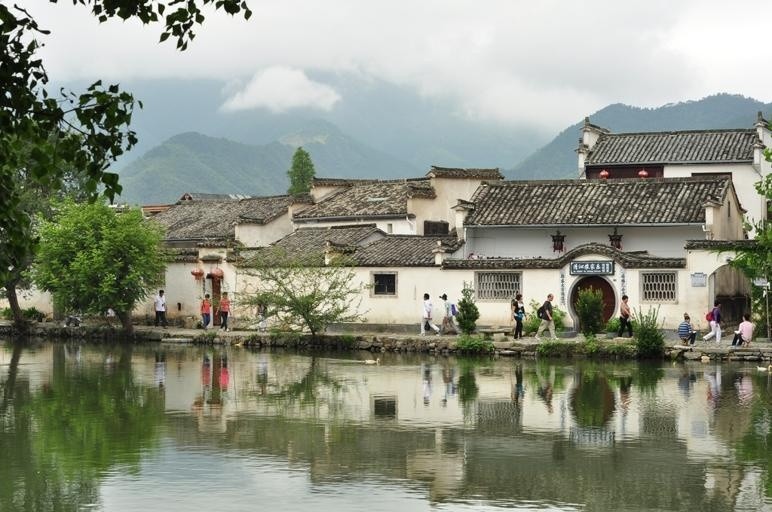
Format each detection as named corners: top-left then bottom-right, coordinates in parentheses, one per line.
top-left (438, 292), bottom-right (461, 337)
top-left (255, 300), bottom-right (268, 334)
top-left (62, 313), bottom-right (80, 328)
top-left (510, 361), bottom-right (529, 411)
top-left (509, 293), bottom-right (529, 342)
top-left (153, 348), bottom-right (167, 391)
top-left (257, 348), bottom-right (268, 395)
top-left (677, 316), bottom-right (699, 347)
top-left (198, 293), bottom-right (212, 331)
top-left (617, 376), bottom-right (633, 417)
top-left (416, 293), bottom-right (440, 337)
top-left (218, 350), bottom-right (229, 393)
top-left (534, 293), bottom-right (560, 342)
top-left (703, 299), bottom-right (726, 349)
top-left (702, 369), bottom-right (725, 414)
top-left (617, 295), bottom-right (634, 339)
top-left (729, 312), bottom-right (754, 350)
top-left (676, 369), bottom-right (697, 403)
top-left (201, 349), bottom-right (213, 395)
top-left (732, 372), bottom-right (754, 411)
top-left (534, 363), bottom-right (557, 414)
top-left (153, 289), bottom-right (169, 330)
top-left (440, 365), bottom-right (456, 409)
top-left (214, 291), bottom-right (232, 332)
top-left (418, 358), bottom-right (432, 408)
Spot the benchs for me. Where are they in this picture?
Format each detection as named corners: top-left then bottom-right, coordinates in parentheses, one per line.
top-left (479, 328), bottom-right (513, 339)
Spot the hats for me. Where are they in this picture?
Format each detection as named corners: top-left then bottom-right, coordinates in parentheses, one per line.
top-left (440, 293), bottom-right (447, 298)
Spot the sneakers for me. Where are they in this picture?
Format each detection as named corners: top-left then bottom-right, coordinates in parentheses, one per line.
top-left (690, 343), bottom-right (696, 346)
top-left (702, 338), bottom-right (706, 344)
top-left (219, 328), bottom-right (229, 332)
top-left (535, 334), bottom-right (540, 340)
top-left (551, 338), bottom-right (559, 340)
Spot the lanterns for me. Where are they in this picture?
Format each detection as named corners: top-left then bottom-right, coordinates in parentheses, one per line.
top-left (190, 267), bottom-right (204, 281)
top-left (600, 169), bottom-right (609, 179)
top-left (210, 267), bottom-right (224, 283)
top-left (638, 170), bottom-right (650, 179)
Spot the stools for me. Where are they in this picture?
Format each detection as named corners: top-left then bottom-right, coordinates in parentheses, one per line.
top-left (681, 337), bottom-right (689, 346)
top-left (741, 340), bottom-right (749, 347)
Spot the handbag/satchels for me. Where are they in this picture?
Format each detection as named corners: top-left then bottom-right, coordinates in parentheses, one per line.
top-left (517, 312), bottom-right (523, 318)
top-left (706, 312), bottom-right (713, 321)
top-left (451, 305), bottom-right (456, 314)
top-left (537, 306), bottom-right (545, 318)
top-left (425, 321), bottom-right (430, 330)
top-left (624, 314), bottom-right (629, 318)
